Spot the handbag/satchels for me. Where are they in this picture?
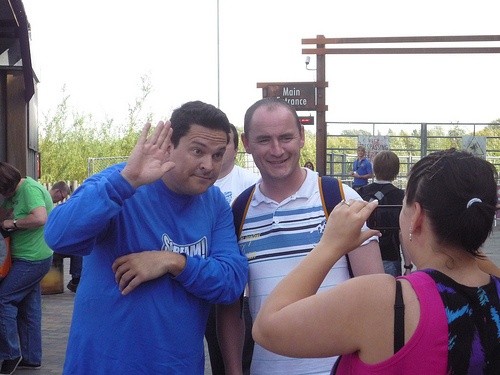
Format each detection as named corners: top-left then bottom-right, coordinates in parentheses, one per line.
top-left (0, 230), bottom-right (12, 279)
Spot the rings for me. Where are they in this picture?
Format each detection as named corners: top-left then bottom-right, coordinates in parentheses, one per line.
top-left (345, 202), bottom-right (351, 207)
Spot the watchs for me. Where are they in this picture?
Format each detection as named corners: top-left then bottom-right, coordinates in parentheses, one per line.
top-left (13, 220), bottom-right (18, 227)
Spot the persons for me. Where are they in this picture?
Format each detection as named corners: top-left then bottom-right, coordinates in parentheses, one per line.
top-left (251, 147), bottom-right (500, 375)
top-left (355, 151), bottom-right (413, 277)
top-left (305, 162), bottom-right (315, 170)
top-left (216, 98), bottom-right (386, 375)
top-left (349, 147), bottom-right (373, 190)
top-left (49, 181), bottom-right (82, 293)
top-left (204, 123), bottom-right (259, 375)
top-left (0, 160), bottom-right (52, 375)
top-left (43, 100), bottom-right (249, 375)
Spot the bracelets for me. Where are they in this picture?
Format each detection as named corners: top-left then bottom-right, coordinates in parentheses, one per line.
top-left (404, 264), bottom-right (413, 269)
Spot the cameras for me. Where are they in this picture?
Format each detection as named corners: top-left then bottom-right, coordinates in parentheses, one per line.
top-left (367, 205), bottom-right (403, 229)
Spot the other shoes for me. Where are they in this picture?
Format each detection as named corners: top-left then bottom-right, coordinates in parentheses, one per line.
top-left (67, 280), bottom-right (79, 292)
top-left (0, 355), bottom-right (22, 375)
top-left (17, 359), bottom-right (40, 369)
